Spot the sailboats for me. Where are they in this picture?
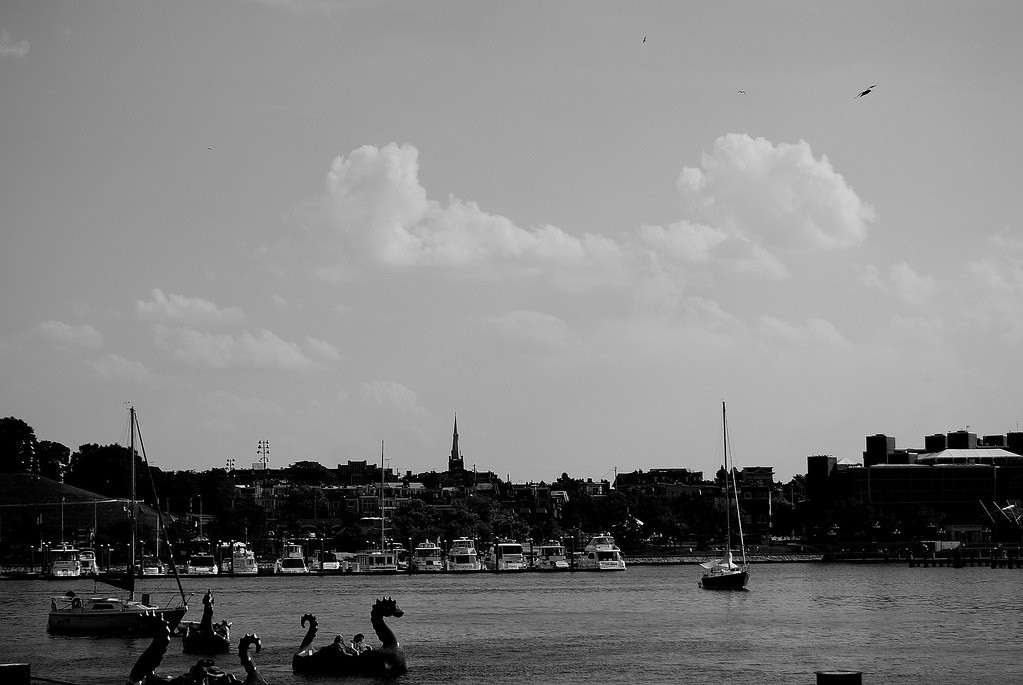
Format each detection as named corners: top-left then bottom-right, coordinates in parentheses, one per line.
top-left (44, 406), bottom-right (191, 638)
top-left (698, 402), bottom-right (752, 590)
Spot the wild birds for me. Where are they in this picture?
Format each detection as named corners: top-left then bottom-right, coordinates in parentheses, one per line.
top-left (641, 36), bottom-right (877, 99)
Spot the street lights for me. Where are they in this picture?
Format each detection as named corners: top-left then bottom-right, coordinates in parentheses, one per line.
top-left (256, 439), bottom-right (271, 529)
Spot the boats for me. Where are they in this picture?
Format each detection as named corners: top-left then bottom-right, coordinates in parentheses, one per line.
top-left (291, 597), bottom-right (409, 681)
top-left (137, 537), bottom-right (569, 574)
top-left (182, 589), bottom-right (232, 657)
top-left (118, 611), bottom-right (269, 684)
top-left (43, 496), bottom-right (83, 581)
top-left (577, 532), bottom-right (627, 571)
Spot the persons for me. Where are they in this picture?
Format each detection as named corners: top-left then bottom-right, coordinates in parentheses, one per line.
top-left (189, 658), bottom-right (225, 685)
top-left (188, 619), bottom-right (230, 639)
top-left (334, 634), bottom-right (356, 657)
top-left (349, 633), bottom-right (373, 656)
